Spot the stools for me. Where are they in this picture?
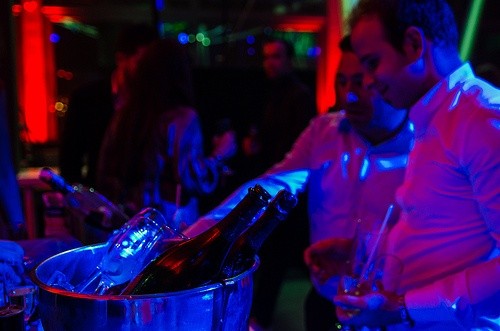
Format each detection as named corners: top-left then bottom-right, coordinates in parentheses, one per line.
top-left (17, 166), bottom-right (88, 239)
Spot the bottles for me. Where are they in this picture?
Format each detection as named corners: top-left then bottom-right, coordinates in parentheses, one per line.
top-left (212, 190), bottom-right (298, 283)
top-left (38, 167), bottom-right (131, 232)
top-left (71, 207), bottom-right (171, 295)
top-left (122, 184), bottom-right (273, 296)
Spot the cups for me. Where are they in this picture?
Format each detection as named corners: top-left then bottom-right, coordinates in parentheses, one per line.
top-left (8, 284), bottom-right (38, 331)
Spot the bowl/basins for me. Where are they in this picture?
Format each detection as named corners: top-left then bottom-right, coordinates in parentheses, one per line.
top-left (30, 239), bottom-right (260, 331)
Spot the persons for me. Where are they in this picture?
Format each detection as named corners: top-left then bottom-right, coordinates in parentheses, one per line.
top-left (0, 0), bottom-right (417, 331)
top-left (303, 0), bottom-right (500, 331)
